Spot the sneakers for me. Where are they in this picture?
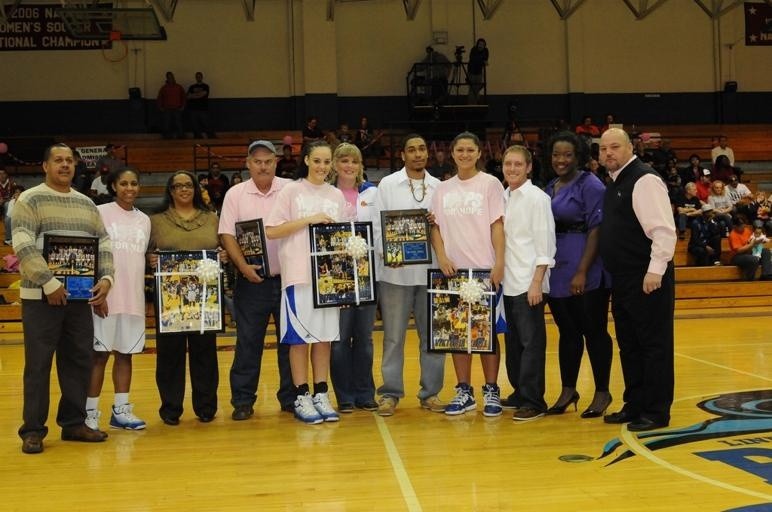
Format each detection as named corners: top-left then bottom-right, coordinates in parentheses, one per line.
top-left (338, 404), bottom-right (354, 412)
top-left (512, 402), bottom-right (546, 419)
top-left (363, 401), bottom-right (377, 410)
top-left (109, 403), bottom-right (145, 429)
top-left (444, 386), bottom-right (477, 414)
top-left (85, 409), bottom-right (100, 431)
top-left (421, 394), bottom-right (447, 412)
top-left (500, 399), bottom-right (519, 409)
top-left (294, 395), bottom-right (323, 424)
top-left (311, 393), bottom-right (339, 421)
top-left (379, 395), bottom-right (398, 415)
top-left (482, 383), bottom-right (503, 416)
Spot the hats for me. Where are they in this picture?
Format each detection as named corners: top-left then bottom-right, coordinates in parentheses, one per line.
top-left (248, 140), bottom-right (275, 153)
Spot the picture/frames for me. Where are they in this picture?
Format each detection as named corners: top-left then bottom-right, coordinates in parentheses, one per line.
top-left (425, 264), bottom-right (499, 355)
top-left (379, 206), bottom-right (436, 267)
top-left (232, 217), bottom-right (273, 280)
top-left (306, 219), bottom-right (380, 310)
top-left (41, 231), bottom-right (102, 312)
top-left (149, 244), bottom-right (228, 338)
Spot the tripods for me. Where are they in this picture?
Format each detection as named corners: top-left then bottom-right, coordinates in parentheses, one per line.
top-left (446, 61), bottom-right (479, 104)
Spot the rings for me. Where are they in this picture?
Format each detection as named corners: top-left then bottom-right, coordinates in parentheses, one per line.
top-left (576, 289), bottom-right (580, 293)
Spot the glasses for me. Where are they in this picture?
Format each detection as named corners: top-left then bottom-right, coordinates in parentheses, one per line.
top-left (170, 182), bottom-right (193, 187)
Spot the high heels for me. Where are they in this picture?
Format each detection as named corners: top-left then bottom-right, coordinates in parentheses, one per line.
top-left (581, 395), bottom-right (612, 418)
top-left (546, 392), bottom-right (579, 414)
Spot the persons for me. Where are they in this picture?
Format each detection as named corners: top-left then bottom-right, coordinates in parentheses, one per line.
top-left (373, 132), bottom-right (448, 415)
top-left (423, 46), bottom-right (452, 106)
top-left (544, 130), bottom-right (615, 417)
top-left (430, 133), bottom-right (507, 417)
top-left (217, 140), bottom-right (298, 421)
top-left (84, 167), bottom-right (152, 431)
top-left (466, 38), bottom-right (490, 104)
top-left (326, 143), bottom-right (378, 411)
top-left (157, 70), bottom-right (211, 139)
top-left (10, 142), bottom-right (115, 453)
top-left (0, 115), bottom-right (771, 278)
top-left (265, 140), bottom-right (347, 425)
top-left (501, 144), bottom-right (558, 421)
top-left (597, 128), bottom-right (678, 432)
top-left (150, 170), bottom-right (219, 425)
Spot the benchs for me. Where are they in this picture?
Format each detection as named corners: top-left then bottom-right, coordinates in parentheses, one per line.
top-left (0, 120), bottom-right (772, 324)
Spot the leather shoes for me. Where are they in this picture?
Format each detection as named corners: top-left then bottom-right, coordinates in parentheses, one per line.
top-left (232, 407), bottom-right (250, 420)
top-left (627, 417), bottom-right (667, 430)
top-left (22, 436), bottom-right (42, 453)
top-left (164, 417), bottom-right (177, 424)
top-left (604, 409), bottom-right (631, 423)
top-left (62, 422), bottom-right (107, 442)
